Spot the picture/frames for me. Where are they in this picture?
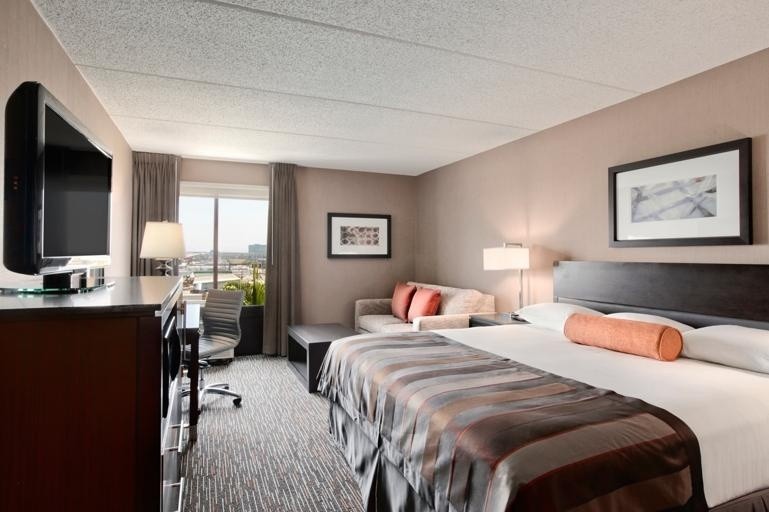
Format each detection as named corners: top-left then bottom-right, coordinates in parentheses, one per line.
top-left (326, 211), bottom-right (393, 260)
top-left (606, 137), bottom-right (755, 249)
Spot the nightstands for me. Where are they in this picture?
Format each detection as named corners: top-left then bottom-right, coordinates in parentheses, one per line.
top-left (467, 312), bottom-right (528, 328)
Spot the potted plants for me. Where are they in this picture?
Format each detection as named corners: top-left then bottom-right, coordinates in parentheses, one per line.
top-left (223, 260), bottom-right (265, 358)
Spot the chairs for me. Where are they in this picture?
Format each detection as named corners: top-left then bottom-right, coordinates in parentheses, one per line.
top-left (175, 287), bottom-right (247, 420)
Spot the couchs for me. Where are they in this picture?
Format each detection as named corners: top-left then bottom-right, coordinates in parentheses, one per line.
top-left (353, 279), bottom-right (498, 333)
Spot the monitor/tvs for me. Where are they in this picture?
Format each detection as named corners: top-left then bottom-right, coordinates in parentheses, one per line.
top-left (3, 81), bottom-right (115, 293)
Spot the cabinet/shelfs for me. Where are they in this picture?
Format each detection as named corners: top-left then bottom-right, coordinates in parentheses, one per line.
top-left (2, 272), bottom-right (195, 512)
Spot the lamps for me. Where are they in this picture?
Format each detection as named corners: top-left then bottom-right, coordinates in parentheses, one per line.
top-left (137, 218), bottom-right (189, 277)
top-left (481, 241), bottom-right (530, 310)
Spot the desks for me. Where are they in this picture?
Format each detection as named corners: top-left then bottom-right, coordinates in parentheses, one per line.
top-left (179, 328), bottom-right (203, 444)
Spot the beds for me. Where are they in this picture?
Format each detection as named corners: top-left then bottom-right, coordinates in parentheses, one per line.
top-left (312, 258), bottom-right (769, 511)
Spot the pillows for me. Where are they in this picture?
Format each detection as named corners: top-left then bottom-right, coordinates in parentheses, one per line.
top-left (512, 301), bottom-right (608, 331)
top-left (677, 324), bottom-right (769, 377)
top-left (602, 311), bottom-right (697, 339)
top-left (563, 311), bottom-right (684, 362)
top-left (406, 286), bottom-right (442, 325)
top-left (389, 281), bottom-right (417, 323)
